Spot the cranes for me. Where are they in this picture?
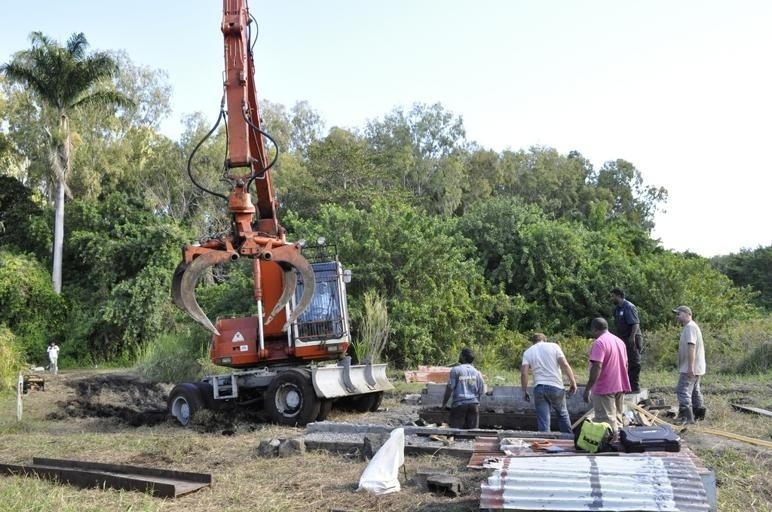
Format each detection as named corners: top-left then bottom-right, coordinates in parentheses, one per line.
top-left (166, 1), bottom-right (396, 427)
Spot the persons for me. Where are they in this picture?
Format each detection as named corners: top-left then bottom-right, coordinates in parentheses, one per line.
top-left (46, 340), bottom-right (61, 375)
top-left (609, 288), bottom-right (644, 394)
top-left (520, 332), bottom-right (578, 433)
top-left (442, 348), bottom-right (488, 428)
top-left (308, 282), bottom-right (340, 321)
top-left (671, 305), bottom-right (707, 424)
top-left (583, 317), bottom-right (633, 443)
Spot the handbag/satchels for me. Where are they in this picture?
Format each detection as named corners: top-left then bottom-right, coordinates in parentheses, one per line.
top-left (574, 418), bottom-right (613, 453)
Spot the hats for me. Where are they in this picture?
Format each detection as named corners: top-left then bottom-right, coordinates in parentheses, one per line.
top-left (671, 305), bottom-right (693, 317)
